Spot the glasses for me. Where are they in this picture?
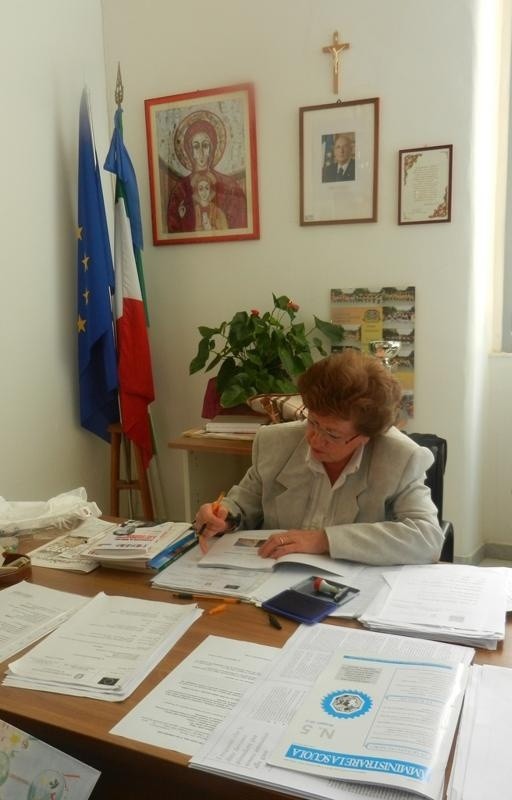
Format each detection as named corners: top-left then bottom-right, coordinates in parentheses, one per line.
top-left (307, 415), bottom-right (360, 446)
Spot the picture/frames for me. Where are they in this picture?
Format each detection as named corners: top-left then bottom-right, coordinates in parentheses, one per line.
top-left (144, 82), bottom-right (259, 246)
top-left (300, 97), bottom-right (380, 227)
top-left (398, 144), bottom-right (452, 225)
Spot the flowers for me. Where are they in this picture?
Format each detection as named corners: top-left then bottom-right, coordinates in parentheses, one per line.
top-left (189, 293), bottom-right (344, 408)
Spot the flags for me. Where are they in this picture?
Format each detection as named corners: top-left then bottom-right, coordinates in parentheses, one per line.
top-left (78, 87), bottom-right (119, 444)
top-left (102, 106), bottom-right (155, 470)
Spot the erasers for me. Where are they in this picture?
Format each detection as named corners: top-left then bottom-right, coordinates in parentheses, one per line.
top-left (114, 527), bottom-right (135, 535)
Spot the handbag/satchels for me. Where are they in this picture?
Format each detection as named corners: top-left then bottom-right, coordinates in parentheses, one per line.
top-left (246, 392), bottom-right (309, 424)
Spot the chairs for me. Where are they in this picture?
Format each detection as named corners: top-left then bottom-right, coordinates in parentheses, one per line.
top-left (399, 430), bottom-right (454, 562)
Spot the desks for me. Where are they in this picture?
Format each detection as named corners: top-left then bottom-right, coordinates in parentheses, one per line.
top-left (168, 417), bottom-right (275, 521)
top-left (1, 515), bottom-right (512, 800)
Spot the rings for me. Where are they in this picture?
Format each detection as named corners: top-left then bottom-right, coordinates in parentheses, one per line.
top-left (280, 537), bottom-right (285, 544)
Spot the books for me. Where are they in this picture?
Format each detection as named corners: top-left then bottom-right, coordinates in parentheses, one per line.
top-left (199, 530), bottom-right (347, 576)
top-left (206, 415), bottom-right (271, 433)
top-left (29, 516), bottom-right (199, 574)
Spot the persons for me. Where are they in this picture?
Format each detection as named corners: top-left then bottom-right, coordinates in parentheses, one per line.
top-left (324, 135), bottom-right (354, 182)
top-left (194, 352), bottom-right (445, 567)
top-left (177, 171), bottom-right (229, 231)
top-left (167, 110), bottom-right (247, 233)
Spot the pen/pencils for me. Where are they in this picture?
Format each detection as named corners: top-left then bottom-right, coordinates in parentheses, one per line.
top-left (172, 594), bottom-right (241, 604)
top-left (196, 495), bottom-right (224, 538)
